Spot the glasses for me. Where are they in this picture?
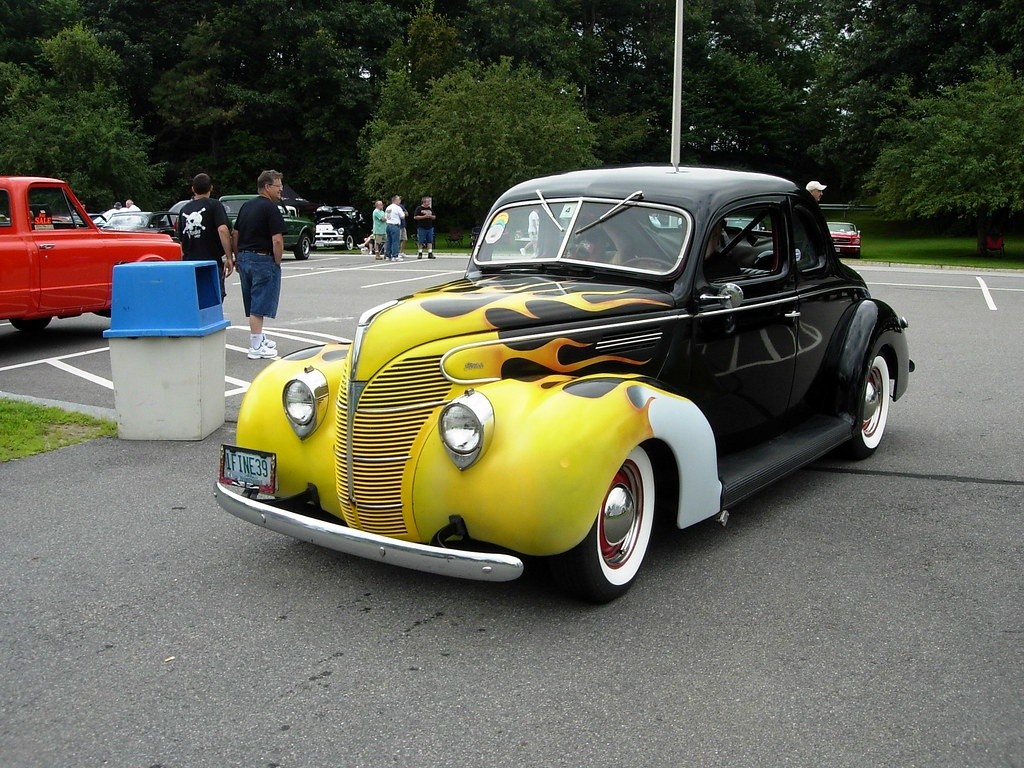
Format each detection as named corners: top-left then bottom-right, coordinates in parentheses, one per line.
top-left (268, 183), bottom-right (283, 190)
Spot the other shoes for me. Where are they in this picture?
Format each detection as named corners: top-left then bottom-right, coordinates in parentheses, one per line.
top-left (386, 257), bottom-right (391, 261)
top-left (391, 257), bottom-right (403, 262)
top-left (369, 251), bottom-right (372, 254)
top-left (428, 253), bottom-right (436, 259)
top-left (398, 253), bottom-right (407, 258)
top-left (417, 252), bottom-right (422, 259)
top-left (357, 244), bottom-right (365, 248)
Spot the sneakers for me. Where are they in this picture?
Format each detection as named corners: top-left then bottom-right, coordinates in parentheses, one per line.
top-left (261, 333), bottom-right (276, 349)
top-left (247, 342), bottom-right (277, 359)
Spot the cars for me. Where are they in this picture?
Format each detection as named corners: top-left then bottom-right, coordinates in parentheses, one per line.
top-left (826, 221), bottom-right (862, 259)
top-left (0, 213), bottom-right (108, 228)
top-left (314, 203), bottom-right (372, 250)
top-left (213, 165), bottom-right (916, 607)
top-left (100, 210), bottom-right (181, 244)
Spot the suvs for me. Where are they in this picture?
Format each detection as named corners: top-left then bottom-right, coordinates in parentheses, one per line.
top-left (218, 195), bottom-right (317, 260)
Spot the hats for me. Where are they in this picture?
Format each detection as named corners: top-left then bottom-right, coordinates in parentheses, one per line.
top-left (806, 181), bottom-right (827, 191)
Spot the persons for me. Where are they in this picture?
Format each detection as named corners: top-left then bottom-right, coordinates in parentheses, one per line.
top-left (177, 173), bottom-right (233, 305)
top-left (79, 200), bottom-right (86, 209)
top-left (806, 181), bottom-right (827, 202)
top-left (114, 199), bottom-right (141, 211)
top-left (373, 195), bottom-right (408, 261)
top-left (703, 218), bottom-right (740, 279)
top-left (414, 197), bottom-right (436, 259)
top-left (231, 170), bottom-right (288, 359)
top-left (520, 205), bottom-right (540, 258)
top-left (565, 212), bottom-right (636, 265)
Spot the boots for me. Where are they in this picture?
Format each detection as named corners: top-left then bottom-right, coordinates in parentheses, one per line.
top-left (384, 251), bottom-right (387, 259)
top-left (375, 251), bottom-right (382, 260)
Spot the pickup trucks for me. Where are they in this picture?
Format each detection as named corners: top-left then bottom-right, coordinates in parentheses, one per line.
top-left (0, 176), bottom-right (184, 333)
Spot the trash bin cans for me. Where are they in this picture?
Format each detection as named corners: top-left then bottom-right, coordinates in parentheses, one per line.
top-left (101, 260), bottom-right (232, 442)
